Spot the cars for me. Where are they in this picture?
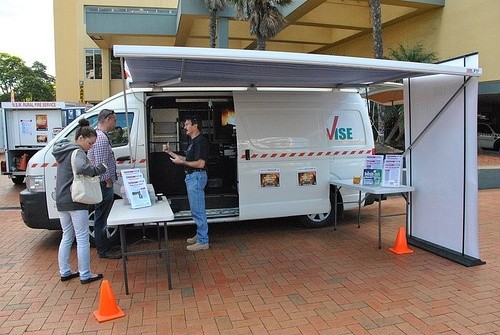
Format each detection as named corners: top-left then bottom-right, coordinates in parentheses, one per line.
top-left (477, 121), bottom-right (500, 152)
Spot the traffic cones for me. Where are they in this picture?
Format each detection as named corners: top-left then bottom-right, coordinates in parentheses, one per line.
top-left (91, 279), bottom-right (127, 322)
top-left (387, 225), bottom-right (414, 256)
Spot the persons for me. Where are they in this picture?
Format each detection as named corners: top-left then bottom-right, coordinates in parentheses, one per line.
top-left (52, 118), bottom-right (108, 285)
top-left (86, 109), bottom-right (127, 259)
top-left (163, 113), bottom-right (210, 252)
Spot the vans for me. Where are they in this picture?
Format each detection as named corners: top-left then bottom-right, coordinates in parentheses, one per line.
top-left (20, 86), bottom-right (377, 247)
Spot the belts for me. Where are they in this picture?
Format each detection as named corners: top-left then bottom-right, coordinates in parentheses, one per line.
top-left (192, 169), bottom-right (202, 172)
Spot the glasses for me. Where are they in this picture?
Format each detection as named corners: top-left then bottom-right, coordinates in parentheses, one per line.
top-left (111, 119), bottom-right (117, 123)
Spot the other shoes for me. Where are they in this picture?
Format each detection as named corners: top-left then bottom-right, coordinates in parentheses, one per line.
top-left (187, 235), bottom-right (197, 244)
top-left (61, 271), bottom-right (80, 282)
top-left (186, 242), bottom-right (209, 252)
top-left (98, 248), bottom-right (122, 259)
top-left (109, 247), bottom-right (122, 252)
top-left (80, 273), bottom-right (103, 284)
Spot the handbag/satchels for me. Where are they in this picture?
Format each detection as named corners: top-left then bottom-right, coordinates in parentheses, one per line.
top-left (71, 148), bottom-right (103, 205)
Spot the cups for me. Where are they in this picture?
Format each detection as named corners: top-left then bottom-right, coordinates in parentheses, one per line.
top-left (156, 194), bottom-right (163, 201)
top-left (353, 175), bottom-right (361, 185)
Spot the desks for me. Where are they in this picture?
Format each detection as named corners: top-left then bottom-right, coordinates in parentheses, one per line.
top-left (329, 178), bottom-right (416, 252)
top-left (107, 196), bottom-right (175, 295)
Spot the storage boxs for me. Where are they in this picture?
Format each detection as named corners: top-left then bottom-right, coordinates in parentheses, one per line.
top-left (208, 178), bottom-right (224, 188)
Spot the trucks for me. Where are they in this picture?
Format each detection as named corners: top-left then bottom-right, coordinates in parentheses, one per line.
top-left (0, 101), bottom-right (94, 186)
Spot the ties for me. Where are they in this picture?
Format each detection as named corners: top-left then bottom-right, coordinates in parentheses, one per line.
top-left (99, 130), bottom-right (118, 181)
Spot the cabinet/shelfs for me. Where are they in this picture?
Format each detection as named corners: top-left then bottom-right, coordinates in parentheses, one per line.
top-left (179, 109), bottom-right (214, 146)
top-left (149, 108), bottom-right (180, 153)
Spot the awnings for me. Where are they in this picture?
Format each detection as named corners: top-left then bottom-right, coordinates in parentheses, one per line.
top-left (112, 44), bottom-right (482, 168)
